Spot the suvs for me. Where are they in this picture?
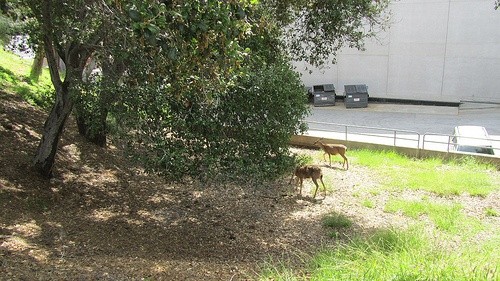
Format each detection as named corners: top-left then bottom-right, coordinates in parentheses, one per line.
top-left (450, 125), bottom-right (495, 154)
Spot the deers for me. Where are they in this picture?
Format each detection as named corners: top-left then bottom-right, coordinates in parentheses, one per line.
top-left (313, 137), bottom-right (349, 170)
top-left (294, 162), bottom-right (327, 198)
